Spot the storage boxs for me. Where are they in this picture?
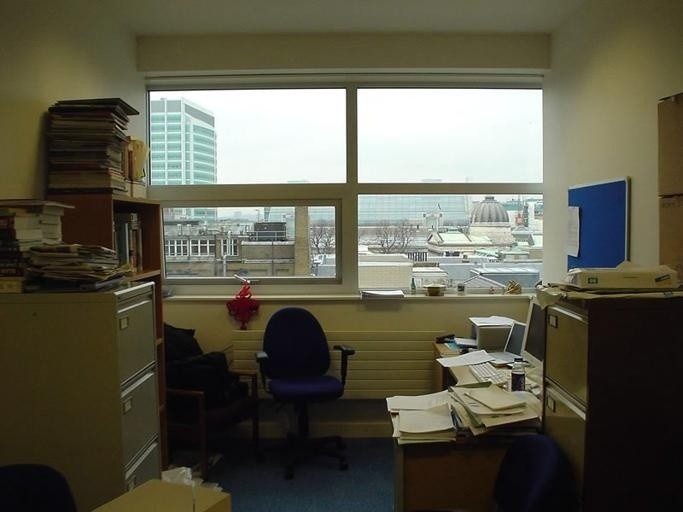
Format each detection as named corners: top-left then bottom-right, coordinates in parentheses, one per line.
top-left (656, 90), bottom-right (683, 273)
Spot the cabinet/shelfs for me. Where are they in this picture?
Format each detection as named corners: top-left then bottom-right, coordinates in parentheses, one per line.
top-left (0, 193), bottom-right (166, 512)
top-left (389, 286), bottom-right (683, 512)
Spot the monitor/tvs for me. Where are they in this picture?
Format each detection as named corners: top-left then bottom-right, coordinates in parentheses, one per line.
top-left (520, 295), bottom-right (554, 375)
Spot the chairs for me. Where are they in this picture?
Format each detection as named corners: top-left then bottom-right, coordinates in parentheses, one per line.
top-left (163, 308), bottom-right (355, 480)
top-left (492, 434), bottom-right (565, 512)
top-left (0, 464), bottom-right (77, 511)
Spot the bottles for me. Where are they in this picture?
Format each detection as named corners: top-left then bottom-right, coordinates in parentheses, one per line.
top-left (510, 357), bottom-right (525, 391)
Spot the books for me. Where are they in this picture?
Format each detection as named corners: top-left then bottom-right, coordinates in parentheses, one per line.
top-left (45, 97), bottom-right (148, 199)
top-left (0, 196), bottom-right (130, 291)
top-left (115, 212), bottom-right (145, 273)
top-left (384, 383), bottom-right (545, 446)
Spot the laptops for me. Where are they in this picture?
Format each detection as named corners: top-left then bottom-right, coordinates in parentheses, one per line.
top-left (487, 321), bottom-right (526, 368)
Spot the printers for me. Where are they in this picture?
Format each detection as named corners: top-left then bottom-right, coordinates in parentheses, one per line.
top-left (454, 316), bottom-right (516, 351)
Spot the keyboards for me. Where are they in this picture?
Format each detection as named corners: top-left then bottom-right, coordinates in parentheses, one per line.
top-left (469, 360), bottom-right (507, 388)
